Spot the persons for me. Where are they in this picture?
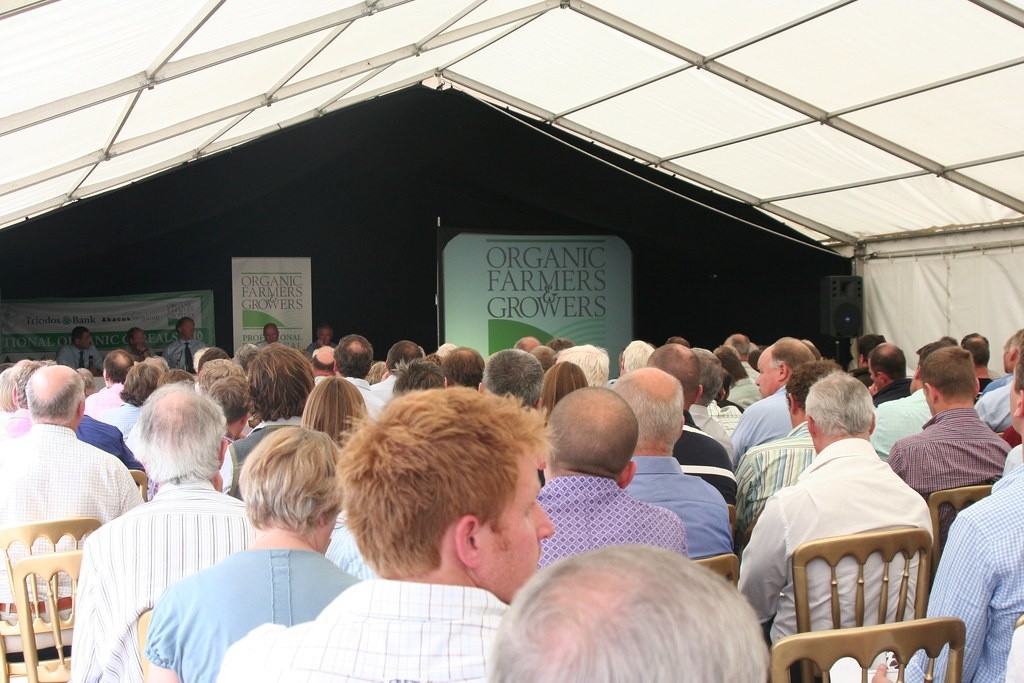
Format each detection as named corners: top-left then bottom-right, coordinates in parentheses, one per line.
top-left (212, 388), bottom-right (555, 682)
top-left (613, 367), bottom-right (737, 560)
top-left (70, 384), bottom-right (260, 681)
top-left (735, 371), bottom-right (933, 650)
top-left (1, 316), bottom-right (1024, 682)
top-left (531, 387), bottom-right (688, 571)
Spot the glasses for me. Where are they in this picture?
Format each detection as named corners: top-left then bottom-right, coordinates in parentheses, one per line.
top-left (222, 432), bottom-right (235, 445)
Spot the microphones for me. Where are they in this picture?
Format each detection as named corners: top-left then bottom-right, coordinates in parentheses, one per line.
top-left (89, 355), bottom-right (93, 370)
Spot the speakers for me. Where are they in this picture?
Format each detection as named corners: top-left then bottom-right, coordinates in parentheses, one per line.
top-left (820, 275), bottom-right (863, 336)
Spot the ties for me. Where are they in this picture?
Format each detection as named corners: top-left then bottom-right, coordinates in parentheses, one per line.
top-left (184, 342), bottom-right (196, 374)
top-left (79, 351), bottom-right (85, 368)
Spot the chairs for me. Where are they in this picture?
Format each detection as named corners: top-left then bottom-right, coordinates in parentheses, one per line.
top-left (0, 469), bottom-right (1000, 683)
